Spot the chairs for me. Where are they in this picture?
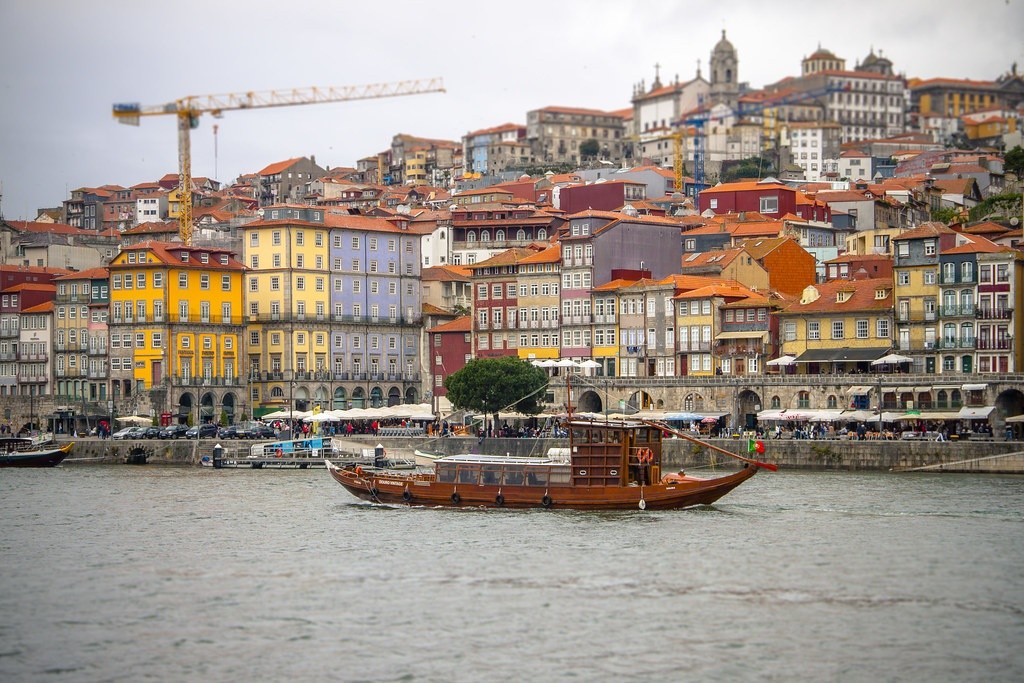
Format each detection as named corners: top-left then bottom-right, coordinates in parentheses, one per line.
top-left (742, 431), bottom-right (899, 440)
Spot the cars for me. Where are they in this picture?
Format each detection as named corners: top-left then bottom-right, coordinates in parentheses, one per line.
top-left (113, 424), bottom-right (277, 440)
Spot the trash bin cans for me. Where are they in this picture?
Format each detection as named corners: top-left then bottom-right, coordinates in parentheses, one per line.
top-left (330, 427), bottom-right (335, 436)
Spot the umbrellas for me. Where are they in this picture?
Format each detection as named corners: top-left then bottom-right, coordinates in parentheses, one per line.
top-left (532, 359), bottom-right (602, 375)
top-left (261, 403), bottom-right (440, 422)
top-left (871, 354), bottom-right (913, 374)
top-left (701, 417), bottom-right (716, 428)
top-left (766, 355), bottom-right (798, 366)
top-left (474, 410), bottom-right (630, 421)
top-left (115, 416), bottom-right (153, 427)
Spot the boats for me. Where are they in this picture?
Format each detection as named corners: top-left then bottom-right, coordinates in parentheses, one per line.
top-left (0, 438), bottom-right (76, 468)
top-left (199, 435), bottom-right (416, 472)
top-left (323, 370), bottom-right (778, 511)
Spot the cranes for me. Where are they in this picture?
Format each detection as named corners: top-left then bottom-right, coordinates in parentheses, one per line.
top-left (672, 82), bottom-right (849, 206)
top-left (110, 77), bottom-right (449, 246)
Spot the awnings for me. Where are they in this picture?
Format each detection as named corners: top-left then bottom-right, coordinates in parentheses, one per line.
top-left (794, 347), bottom-right (890, 362)
top-left (847, 386), bottom-right (873, 395)
top-left (755, 406), bottom-right (997, 422)
top-left (632, 410), bottom-right (704, 420)
top-left (962, 383), bottom-right (988, 390)
top-left (882, 387), bottom-right (932, 392)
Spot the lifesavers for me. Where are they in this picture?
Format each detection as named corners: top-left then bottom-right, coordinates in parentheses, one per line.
top-left (495, 495), bottom-right (504, 505)
top-left (383, 451), bottom-right (386, 457)
top-left (637, 449), bottom-right (646, 462)
top-left (276, 450), bottom-right (282, 458)
top-left (541, 495), bottom-right (553, 509)
top-left (451, 493), bottom-right (460, 504)
top-left (645, 448), bottom-right (654, 462)
top-left (370, 487), bottom-right (379, 497)
top-left (402, 491), bottom-right (412, 501)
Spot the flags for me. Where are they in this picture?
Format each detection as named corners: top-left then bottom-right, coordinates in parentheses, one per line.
top-left (749, 439), bottom-right (765, 453)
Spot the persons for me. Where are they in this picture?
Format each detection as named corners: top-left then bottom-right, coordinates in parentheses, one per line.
top-left (882, 420), bottom-right (993, 442)
top-left (856, 423), bottom-right (866, 440)
top-left (789, 422), bottom-right (848, 440)
top-left (690, 421), bottom-right (700, 434)
top-left (97, 424), bottom-right (110, 439)
top-left (1004, 422), bottom-right (1013, 441)
top-left (270, 420), bottom-right (452, 437)
top-left (738, 424), bottom-right (785, 439)
top-left (820, 368), bottom-right (869, 374)
top-left (476, 422), bottom-right (570, 438)
top-left (882, 365), bottom-right (903, 374)
top-left (870, 428), bottom-right (876, 432)
